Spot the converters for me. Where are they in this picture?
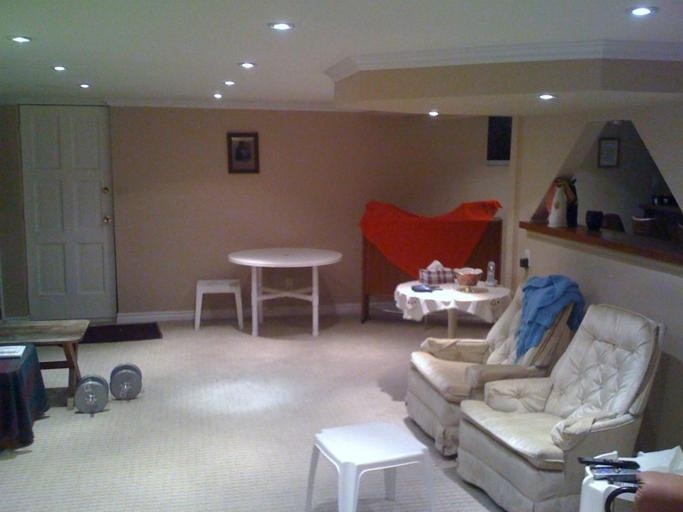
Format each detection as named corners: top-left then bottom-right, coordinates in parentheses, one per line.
top-left (520, 257), bottom-right (528, 268)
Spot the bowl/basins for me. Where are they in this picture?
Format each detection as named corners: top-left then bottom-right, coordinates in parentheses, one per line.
top-left (454, 265), bottom-right (483, 286)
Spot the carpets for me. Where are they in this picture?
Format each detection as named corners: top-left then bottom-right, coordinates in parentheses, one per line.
top-left (59, 321), bottom-right (162, 346)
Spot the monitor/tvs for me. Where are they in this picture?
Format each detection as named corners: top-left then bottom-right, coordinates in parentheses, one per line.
top-left (484, 117), bottom-right (512, 166)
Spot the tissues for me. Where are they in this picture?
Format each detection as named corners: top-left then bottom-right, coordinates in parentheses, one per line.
top-left (419, 259), bottom-right (452, 284)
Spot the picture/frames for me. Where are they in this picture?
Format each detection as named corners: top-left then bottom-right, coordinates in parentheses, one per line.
top-left (599, 136), bottom-right (620, 167)
top-left (227, 131), bottom-right (260, 173)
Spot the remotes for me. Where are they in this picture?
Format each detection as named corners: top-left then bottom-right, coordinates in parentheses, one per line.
top-left (578, 455), bottom-right (639, 469)
top-left (591, 467), bottom-right (640, 479)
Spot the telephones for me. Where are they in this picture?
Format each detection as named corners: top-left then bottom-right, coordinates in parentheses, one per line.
top-left (486, 261), bottom-right (498, 288)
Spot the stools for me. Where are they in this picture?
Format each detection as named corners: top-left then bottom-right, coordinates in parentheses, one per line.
top-left (194, 279), bottom-right (244, 330)
top-left (304, 420), bottom-right (437, 512)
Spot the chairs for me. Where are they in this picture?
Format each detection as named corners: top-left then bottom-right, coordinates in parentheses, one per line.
top-left (457, 304), bottom-right (665, 512)
top-left (406, 284), bottom-right (574, 458)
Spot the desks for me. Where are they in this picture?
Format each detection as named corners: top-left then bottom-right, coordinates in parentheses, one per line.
top-left (0, 342), bottom-right (51, 453)
top-left (228, 248), bottom-right (344, 338)
top-left (394, 280), bottom-right (511, 339)
top-left (360, 217), bottom-right (504, 324)
top-left (0, 319), bottom-right (91, 410)
